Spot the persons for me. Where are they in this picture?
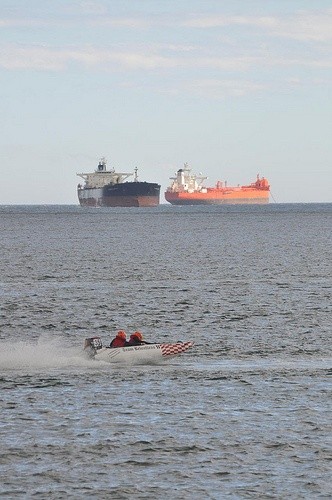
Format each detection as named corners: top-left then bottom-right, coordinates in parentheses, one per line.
top-left (126, 331), bottom-right (147, 346)
top-left (110, 330), bottom-right (126, 348)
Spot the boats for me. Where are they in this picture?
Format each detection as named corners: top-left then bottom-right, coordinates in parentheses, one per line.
top-left (165, 165), bottom-right (271, 204)
top-left (76, 338), bottom-right (194, 364)
top-left (77, 155), bottom-right (161, 207)
